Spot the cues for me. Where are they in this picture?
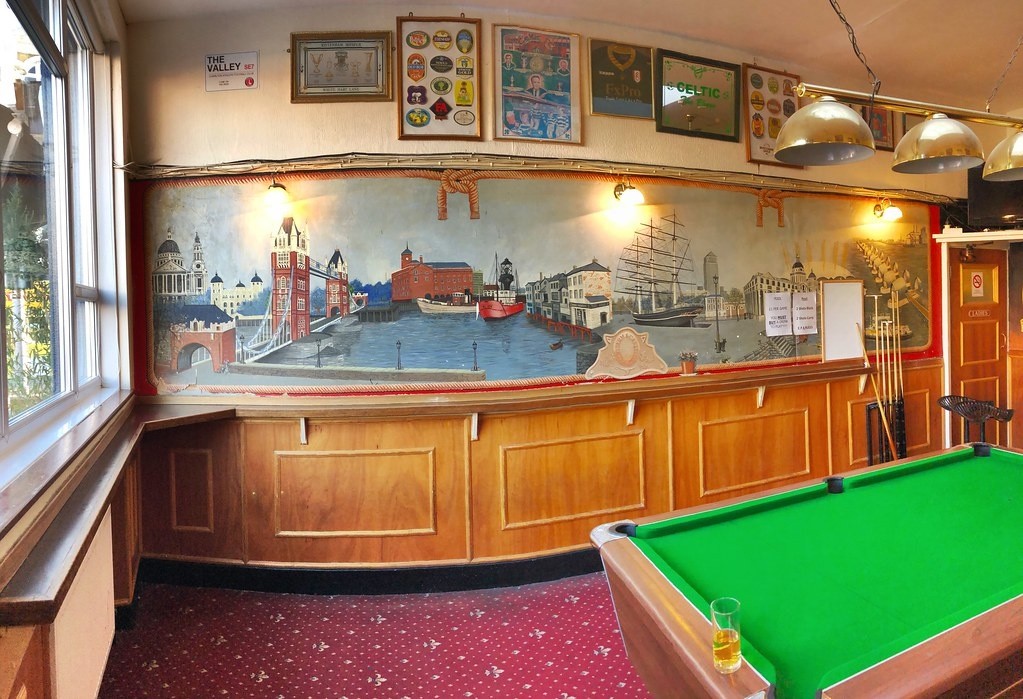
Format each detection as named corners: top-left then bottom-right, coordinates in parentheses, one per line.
top-left (855, 289), bottom-right (907, 462)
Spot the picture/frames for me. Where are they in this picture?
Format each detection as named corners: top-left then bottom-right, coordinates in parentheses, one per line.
top-left (290, 30), bottom-right (392, 103)
top-left (902, 113), bottom-right (927, 136)
top-left (849, 104), bottom-right (895, 152)
top-left (587, 36), bottom-right (655, 120)
top-left (820, 280), bottom-right (866, 364)
top-left (491, 23), bottom-right (584, 145)
top-left (741, 62), bottom-right (804, 170)
top-left (396, 16), bottom-right (482, 141)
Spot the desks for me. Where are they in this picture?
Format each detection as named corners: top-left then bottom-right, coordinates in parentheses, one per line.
top-left (589, 443), bottom-right (1023, 699)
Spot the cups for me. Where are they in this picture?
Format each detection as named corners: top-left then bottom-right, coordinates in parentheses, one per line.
top-left (710, 597), bottom-right (743, 674)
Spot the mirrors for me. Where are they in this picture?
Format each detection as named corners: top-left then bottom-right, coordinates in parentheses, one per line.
top-left (655, 48), bottom-right (741, 142)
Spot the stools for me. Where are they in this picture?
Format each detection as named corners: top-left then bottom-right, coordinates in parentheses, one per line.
top-left (938, 395), bottom-right (1014, 444)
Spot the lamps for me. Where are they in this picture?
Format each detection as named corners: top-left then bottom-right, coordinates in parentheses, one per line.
top-left (614, 174), bottom-right (644, 204)
top-left (7, 110), bottom-right (26, 134)
top-left (874, 197), bottom-right (903, 221)
top-left (773, 0), bottom-right (1023, 181)
